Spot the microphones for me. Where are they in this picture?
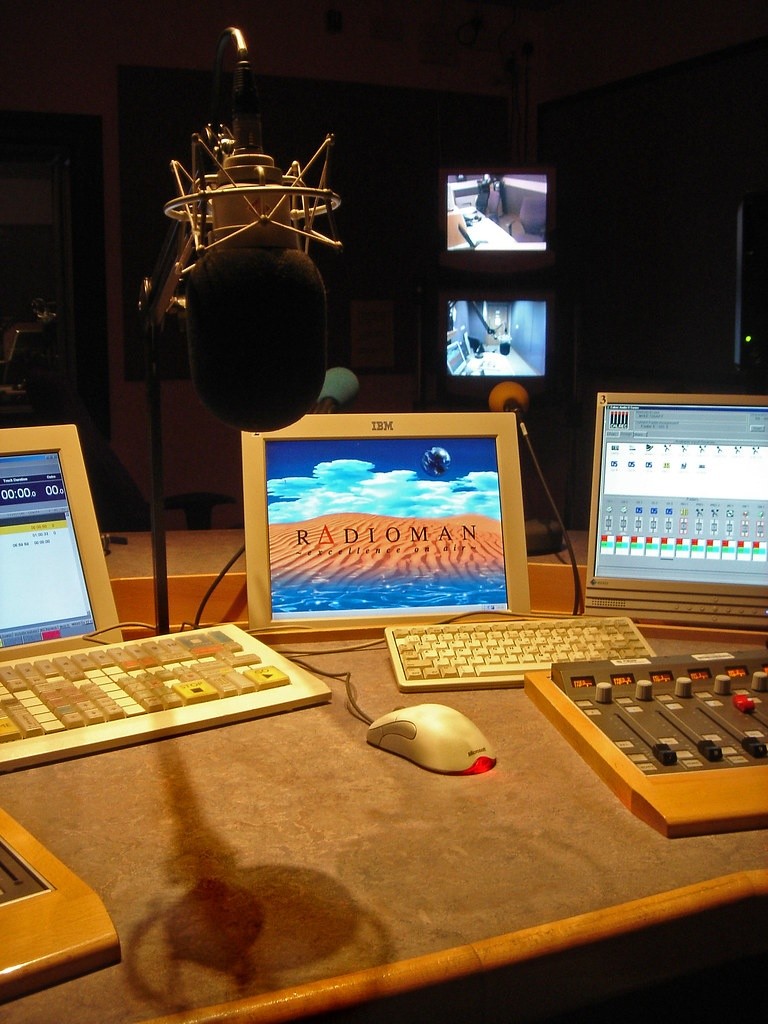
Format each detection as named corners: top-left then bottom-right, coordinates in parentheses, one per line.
top-left (183, 109), bottom-right (328, 435)
top-left (312, 366), bottom-right (360, 415)
top-left (488, 380), bottom-right (581, 616)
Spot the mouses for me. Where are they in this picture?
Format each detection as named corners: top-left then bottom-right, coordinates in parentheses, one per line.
top-left (366, 702), bottom-right (498, 776)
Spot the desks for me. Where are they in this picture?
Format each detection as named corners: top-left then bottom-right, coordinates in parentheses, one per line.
top-left (1, 533), bottom-right (768, 1024)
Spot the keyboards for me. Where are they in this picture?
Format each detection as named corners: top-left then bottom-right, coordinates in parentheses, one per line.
top-left (0, 624), bottom-right (331, 775)
top-left (382, 615), bottom-right (659, 693)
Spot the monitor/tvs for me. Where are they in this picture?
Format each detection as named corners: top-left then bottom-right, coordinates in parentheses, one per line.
top-left (0, 421), bottom-right (123, 664)
top-left (435, 290), bottom-right (562, 405)
top-left (586, 390), bottom-right (768, 630)
top-left (241, 416), bottom-right (533, 641)
top-left (434, 167), bottom-right (558, 276)
